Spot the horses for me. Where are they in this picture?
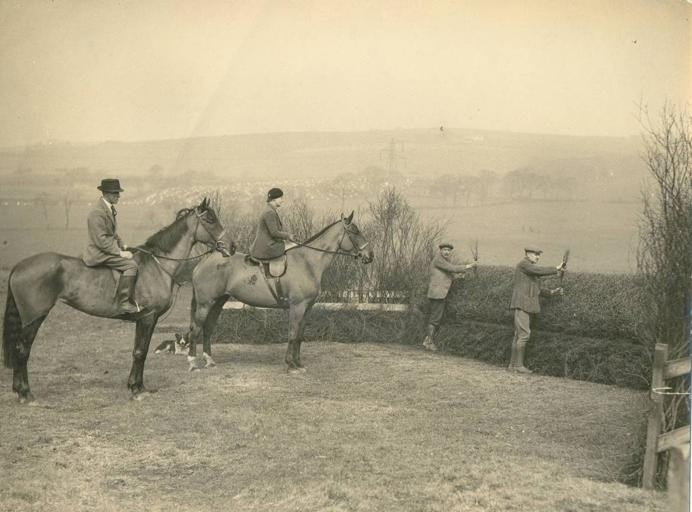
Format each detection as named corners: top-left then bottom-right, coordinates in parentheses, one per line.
top-left (187, 209), bottom-right (374, 375)
top-left (1, 197), bottom-right (238, 408)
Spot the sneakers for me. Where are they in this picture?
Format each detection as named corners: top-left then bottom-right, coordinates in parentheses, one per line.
top-left (422, 335), bottom-right (437, 350)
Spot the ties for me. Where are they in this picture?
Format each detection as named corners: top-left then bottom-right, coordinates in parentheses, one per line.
top-left (111, 205), bottom-right (116, 222)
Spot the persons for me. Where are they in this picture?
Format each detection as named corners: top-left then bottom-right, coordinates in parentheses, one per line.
top-left (80, 178), bottom-right (145, 316)
top-left (419, 242), bottom-right (479, 353)
top-left (507, 245), bottom-right (567, 375)
top-left (248, 187), bottom-right (300, 262)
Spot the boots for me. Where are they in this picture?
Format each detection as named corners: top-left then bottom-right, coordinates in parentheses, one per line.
top-left (508, 343), bottom-right (532, 373)
top-left (116, 273), bottom-right (145, 315)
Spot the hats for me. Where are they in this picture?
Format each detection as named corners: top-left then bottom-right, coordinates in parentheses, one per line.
top-left (524, 246), bottom-right (544, 253)
top-left (97, 178), bottom-right (125, 193)
top-left (439, 242), bottom-right (454, 250)
top-left (266, 188), bottom-right (284, 203)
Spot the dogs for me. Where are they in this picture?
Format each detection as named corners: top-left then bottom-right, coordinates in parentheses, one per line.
top-left (153, 332), bottom-right (190, 355)
